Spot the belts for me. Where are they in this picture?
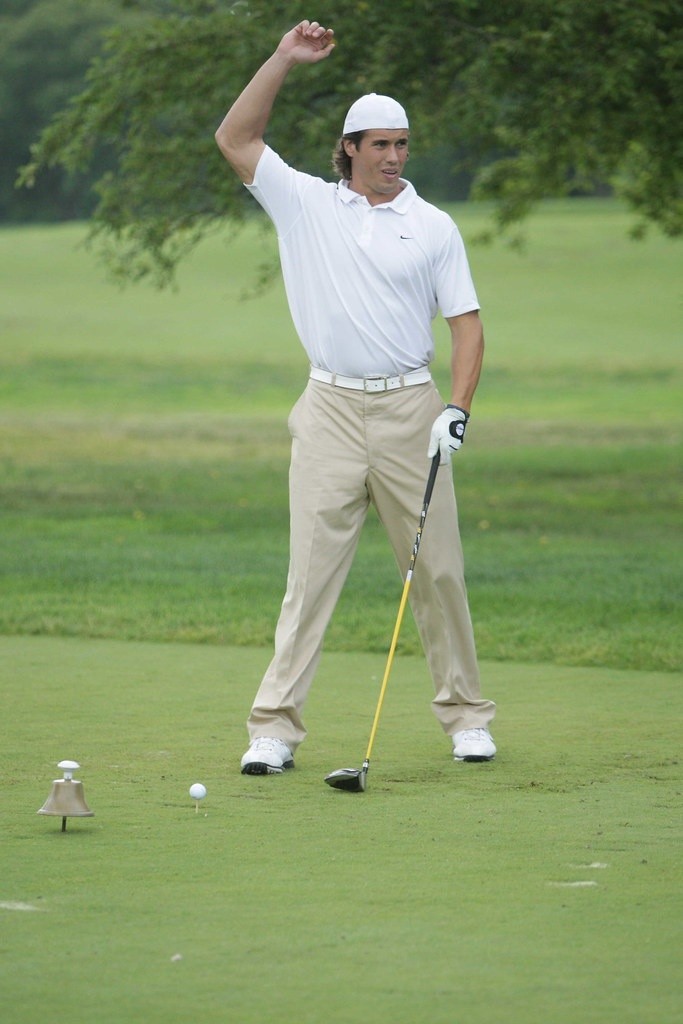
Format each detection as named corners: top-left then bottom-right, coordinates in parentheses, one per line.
top-left (308, 367), bottom-right (431, 394)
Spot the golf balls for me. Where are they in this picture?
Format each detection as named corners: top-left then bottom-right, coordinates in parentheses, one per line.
top-left (189, 783), bottom-right (207, 800)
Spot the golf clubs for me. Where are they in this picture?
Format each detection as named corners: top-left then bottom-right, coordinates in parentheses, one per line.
top-left (323, 452), bottom-right (445, 793)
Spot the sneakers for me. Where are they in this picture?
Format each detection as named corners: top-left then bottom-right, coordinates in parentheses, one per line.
top-left (240, 737), bottom-right (294, 774)
top-left (452, 727), bottom-right (497, 763)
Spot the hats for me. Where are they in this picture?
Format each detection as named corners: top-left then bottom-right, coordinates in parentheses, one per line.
top-left (342, 92), bottom-right (409, 134)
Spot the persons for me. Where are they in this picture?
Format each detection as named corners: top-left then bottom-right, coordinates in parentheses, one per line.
top-left (214, 19), bottom-right (498, 774)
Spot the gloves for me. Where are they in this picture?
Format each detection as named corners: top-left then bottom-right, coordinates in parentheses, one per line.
top-left (427, 404), bottom-right (469, 465)
